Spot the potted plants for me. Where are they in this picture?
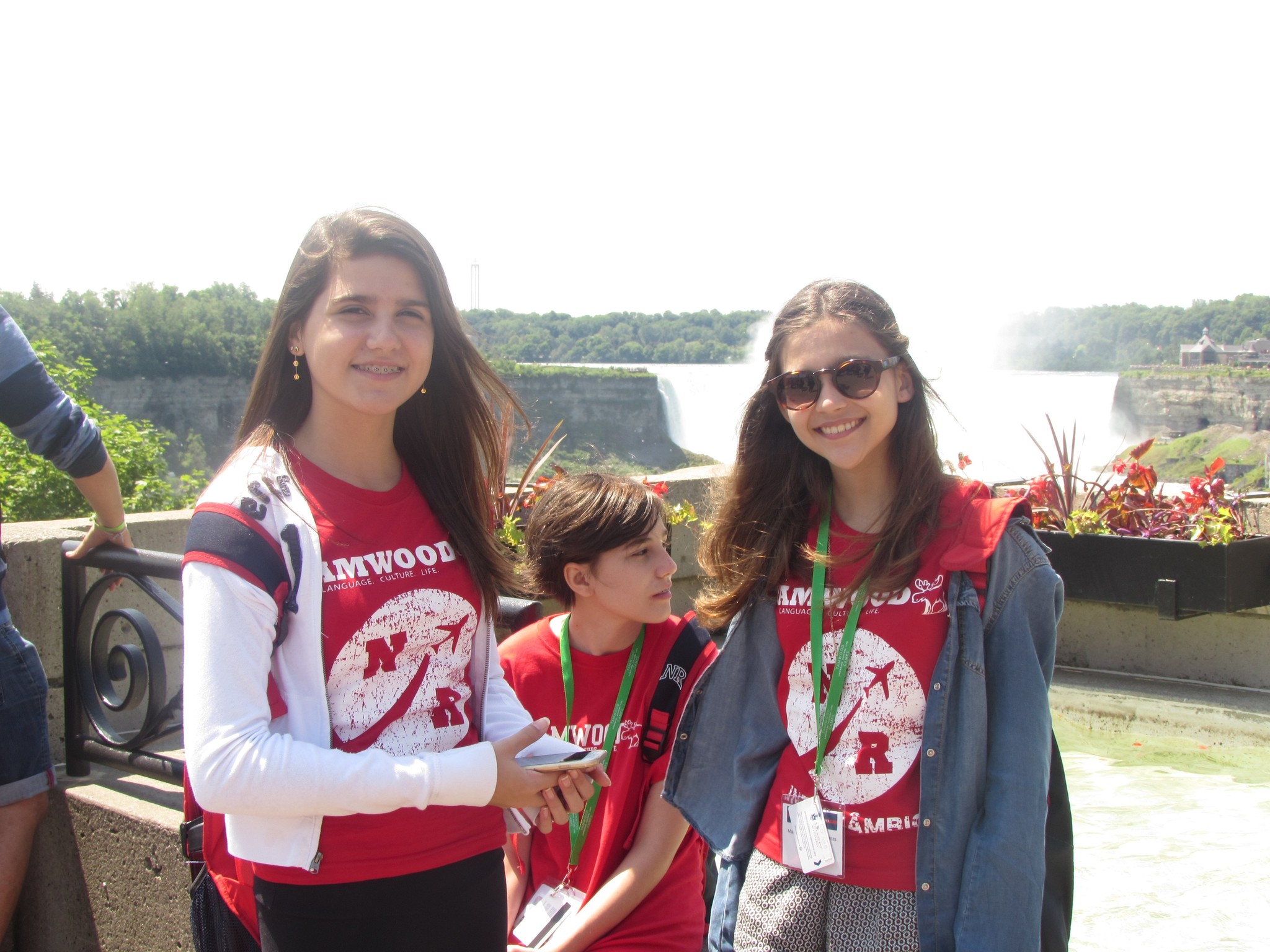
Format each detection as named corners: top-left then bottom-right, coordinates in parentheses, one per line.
top-left (1019, 413), bottom-right (1270, 620)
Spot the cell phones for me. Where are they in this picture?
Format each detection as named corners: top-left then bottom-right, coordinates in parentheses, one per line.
top-left (517, 749), bottom-right (609, 771)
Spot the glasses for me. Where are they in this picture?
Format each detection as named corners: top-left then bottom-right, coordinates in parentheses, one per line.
top-left (764, 354), bottom-right (901, 412)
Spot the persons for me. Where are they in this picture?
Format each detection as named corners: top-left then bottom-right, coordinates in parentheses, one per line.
top-left (660, 279), bottom-right (1063, 952)
top-left (181, 211), bottom-right (611, 952)
top-left (498, 473), bottom-right (717, 952)
top-left (0, 305), bottom-right (136, 946)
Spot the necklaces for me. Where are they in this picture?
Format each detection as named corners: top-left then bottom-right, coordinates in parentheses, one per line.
top-left (829, 544), bottom-right (842, 657)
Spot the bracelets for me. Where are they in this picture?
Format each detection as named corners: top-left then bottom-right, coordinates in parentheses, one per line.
top-left (92, 514), bottom-right (128, 544)
